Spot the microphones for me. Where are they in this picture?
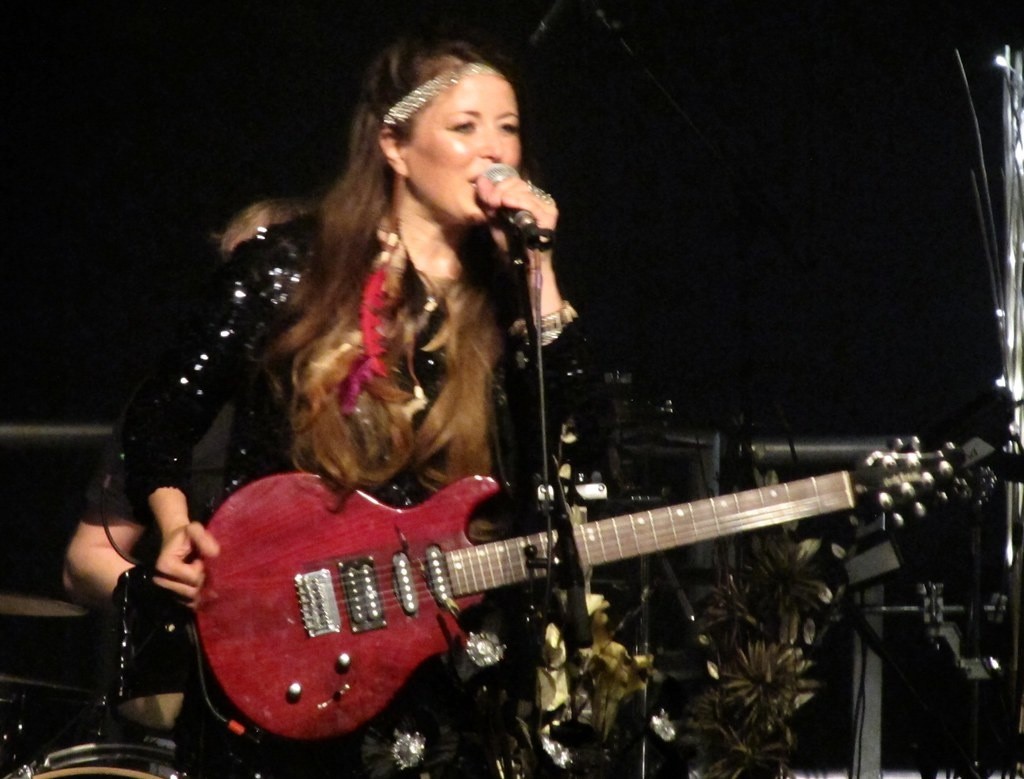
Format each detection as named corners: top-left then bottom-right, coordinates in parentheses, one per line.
top-left (482, 163), bottom-right (542, 238)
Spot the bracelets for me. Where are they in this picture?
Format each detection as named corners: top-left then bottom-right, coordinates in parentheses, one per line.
top-left (541, 301), bottom-right (577, 340)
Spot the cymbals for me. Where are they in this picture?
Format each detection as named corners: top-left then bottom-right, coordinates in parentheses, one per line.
top-left (0, 594), bottom-right (89, 617)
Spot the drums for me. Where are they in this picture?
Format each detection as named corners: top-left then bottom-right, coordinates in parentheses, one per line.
top-left (0, 722), bottom-right (193, 779)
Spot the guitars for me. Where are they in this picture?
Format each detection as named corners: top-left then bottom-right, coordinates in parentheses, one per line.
top-left (187, 434), bottom-right (972, 741)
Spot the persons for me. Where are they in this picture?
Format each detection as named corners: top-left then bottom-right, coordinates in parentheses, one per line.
top-left (130, 41), bottom-right (615, 778)
top-left (61, 199), bottom-right (312, 727)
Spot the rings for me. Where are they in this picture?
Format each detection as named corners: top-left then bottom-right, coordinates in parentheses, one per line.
top-left (530, 183), bottom-right (547, 197)
top-left (542, 194), bottom-right (551, 203)
